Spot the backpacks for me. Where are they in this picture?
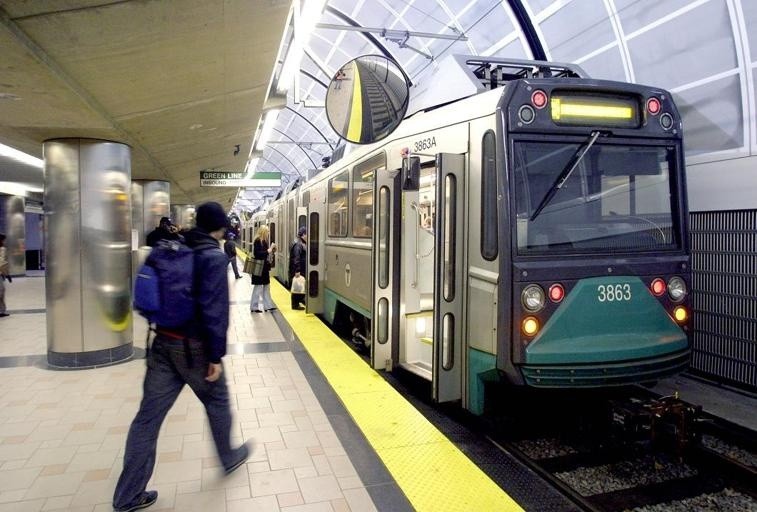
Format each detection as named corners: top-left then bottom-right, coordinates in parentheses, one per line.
top-left (133, 238), bottom-right (219, 327)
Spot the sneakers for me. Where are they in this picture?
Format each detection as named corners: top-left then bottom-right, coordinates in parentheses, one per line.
top-left (113, 491), bottom-right (157, 512)
top-left (224, 446), bottom-right (248, 473)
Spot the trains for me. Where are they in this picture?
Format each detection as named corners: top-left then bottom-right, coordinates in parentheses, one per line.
top-left (234, 54), bottom-right (696, 419)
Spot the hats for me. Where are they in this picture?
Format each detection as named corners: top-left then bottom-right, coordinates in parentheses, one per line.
top-left (227, 233), bottom-right (236, 237)
top-left (196, 202), bottom-right (225, 232)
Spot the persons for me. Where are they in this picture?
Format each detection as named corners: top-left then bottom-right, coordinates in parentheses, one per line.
top-left (110, 201), bottom-right (254, 512)
top-left (224, 231), bottom-right (243, 280)
top-left (250, 225), bottom-right (279, 313)
top-left (288, 225), bottom-right (307, 310)
top-left (0, 234), bottom-right (10, 317)
top-left (143, 215), bottom-right (176, 245)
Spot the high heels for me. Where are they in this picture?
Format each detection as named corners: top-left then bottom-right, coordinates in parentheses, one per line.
top-left (265, 308), bottom-right (276, 312)
top-left (251, 310), bottom-right (262, 313)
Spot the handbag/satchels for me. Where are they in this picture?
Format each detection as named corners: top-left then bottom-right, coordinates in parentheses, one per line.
top-left (243, 257), bottom-right (264, 277)
top-left (291, 275), bottom-right (306, 294)
